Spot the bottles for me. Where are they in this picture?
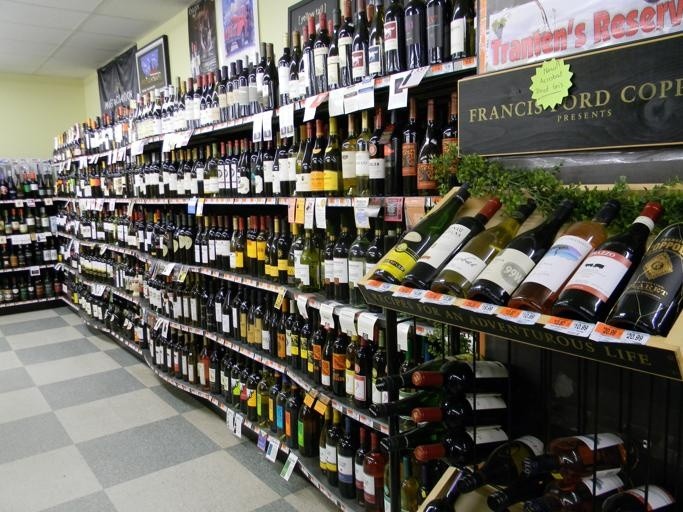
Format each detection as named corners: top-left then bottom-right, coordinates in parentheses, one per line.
top-left (411, 427), bottom-right (509, 467)
top-left (412, 357), bottom-right (507, 394)
top-left (0, 159), bottom-right (59, 306)
top-left (455, 430), bottom-right (681, 511)
top-left (411, 392), bottom-right (506, 426)
top-left (54, 0), bottom-right (473, 512)
top-left (432, 197), bottom-right (682, 336)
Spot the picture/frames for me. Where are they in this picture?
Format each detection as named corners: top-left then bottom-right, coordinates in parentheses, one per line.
top-left (132, 34), bottom-right (170, 94)
top-left (286, 0), bottom-right (341, 48)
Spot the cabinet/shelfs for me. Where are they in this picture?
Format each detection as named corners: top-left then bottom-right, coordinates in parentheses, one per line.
top-left (0, 159), bottom-right (52, 316)
top-left (52, 58), bottom-right (681, 511)
